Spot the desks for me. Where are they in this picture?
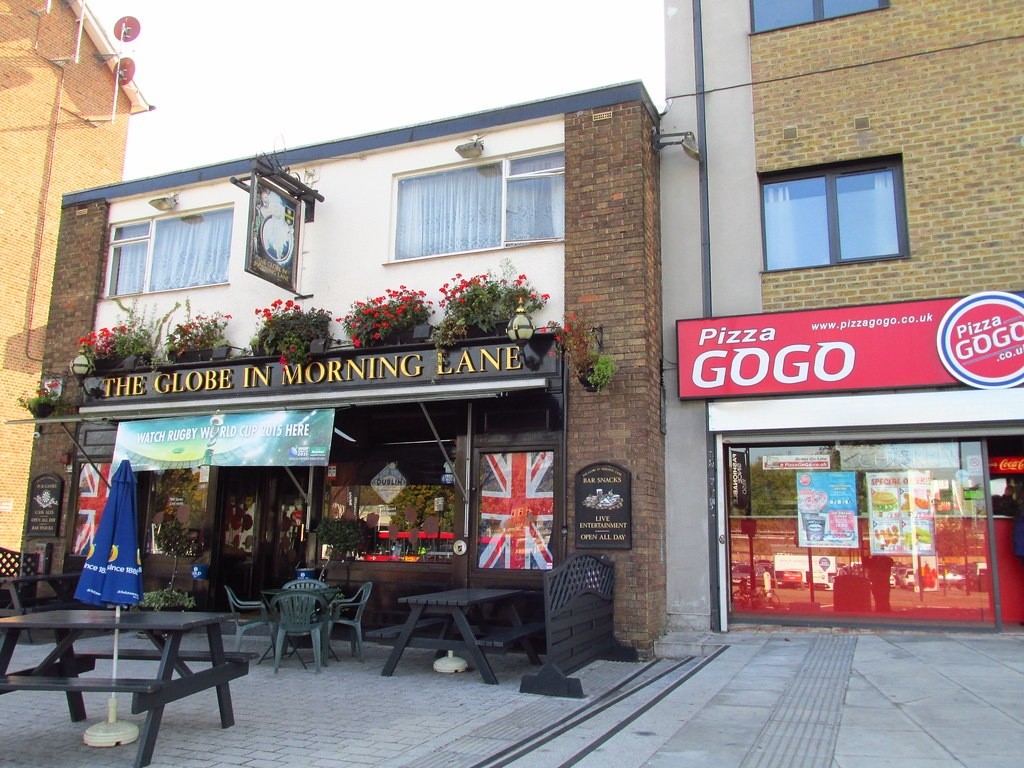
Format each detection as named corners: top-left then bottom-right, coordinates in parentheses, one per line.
top-left (381, 587), bottom-right (542, 685)
top-left (0, 572), bottom-right (82, 644)
top-left (0, 610), bottom-right (249, 768)
top-left (257, 587), bottom-right (339, 670)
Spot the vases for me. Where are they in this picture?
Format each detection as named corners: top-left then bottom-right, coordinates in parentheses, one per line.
top-left (92, 355), bottom-right (148, 368)
top-left (31, 404), bottom-right (55, 418)
top-left (579, 368), bottom-right (610, 393)
top-left (355, 324), bottom-right (433, 349)
top-left (166, 346), bottom-right (229, 363)
top-left (451, 320), bottom-right (511, 340)
top-left (251, 340), bottom-right (310, 356)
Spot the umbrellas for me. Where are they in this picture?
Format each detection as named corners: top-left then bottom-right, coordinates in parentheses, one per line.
top-left (73, 454), bottom-right (145, 747)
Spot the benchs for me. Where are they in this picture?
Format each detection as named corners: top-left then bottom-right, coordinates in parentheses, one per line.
top-left (0, 649), bottom-right (259, 694)
top-left (0, 596), bottom-right (88, 643)
top-left (364, 616), bottom-right (545, 647)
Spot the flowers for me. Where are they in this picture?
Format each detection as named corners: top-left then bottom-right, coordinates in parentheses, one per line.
top-left (16, 273), bottom-right (617, 411)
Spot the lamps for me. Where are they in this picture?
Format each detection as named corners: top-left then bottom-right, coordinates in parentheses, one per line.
top-left (506, 297), bottom-right (534, 370)
top-left (311, 338), bottom-right (341, 354)
top-left (124, 351), bottom-right (154, 371)
top-left (212, 345), bottom-right (249, 360)
top-left (149, 127), bottom-right (702, 211)
top-left (412, 324), bottom-right (440, 338)
top-left (70, 341), bottom-right (104, 399)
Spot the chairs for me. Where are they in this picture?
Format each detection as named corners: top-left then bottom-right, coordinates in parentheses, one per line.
top-left (223, 578), bottom-right (374, 674)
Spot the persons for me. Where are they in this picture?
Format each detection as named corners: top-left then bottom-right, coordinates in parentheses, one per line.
top-left (1007, 484), bottom-right (1024, 561)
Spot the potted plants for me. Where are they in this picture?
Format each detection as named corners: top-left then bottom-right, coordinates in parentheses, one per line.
top-left (136, 519), bottom-right (380, 648)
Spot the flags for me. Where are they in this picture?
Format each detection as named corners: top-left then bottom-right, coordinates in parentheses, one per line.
top-left (477, 450), bottom-right (554, 571)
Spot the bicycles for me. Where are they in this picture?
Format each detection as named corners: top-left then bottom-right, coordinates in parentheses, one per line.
top-left (733, 587), bottom-right (781, 612)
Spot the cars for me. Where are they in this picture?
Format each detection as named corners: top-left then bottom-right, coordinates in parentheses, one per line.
top-left (731, 559), bottom-right (990, 588)
